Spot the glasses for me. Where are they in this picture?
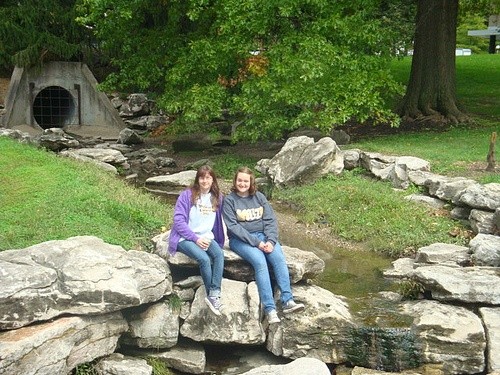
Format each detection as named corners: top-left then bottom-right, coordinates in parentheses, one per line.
top-left (198, 176), bottom-right (211, 180)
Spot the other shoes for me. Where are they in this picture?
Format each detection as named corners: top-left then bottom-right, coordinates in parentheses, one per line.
top-left (282, 300), bottom-right (305, 314)
top-left (266, 308), bottom-right (281, 324)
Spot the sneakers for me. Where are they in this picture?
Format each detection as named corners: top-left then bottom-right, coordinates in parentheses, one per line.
top-left (204, 296), bottom-right (227, 316)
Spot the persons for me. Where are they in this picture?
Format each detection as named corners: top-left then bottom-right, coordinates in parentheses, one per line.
top-left (165, 163), bottom-right (226, 316)
top-left (221, 168), bottom-right (306, 324)
